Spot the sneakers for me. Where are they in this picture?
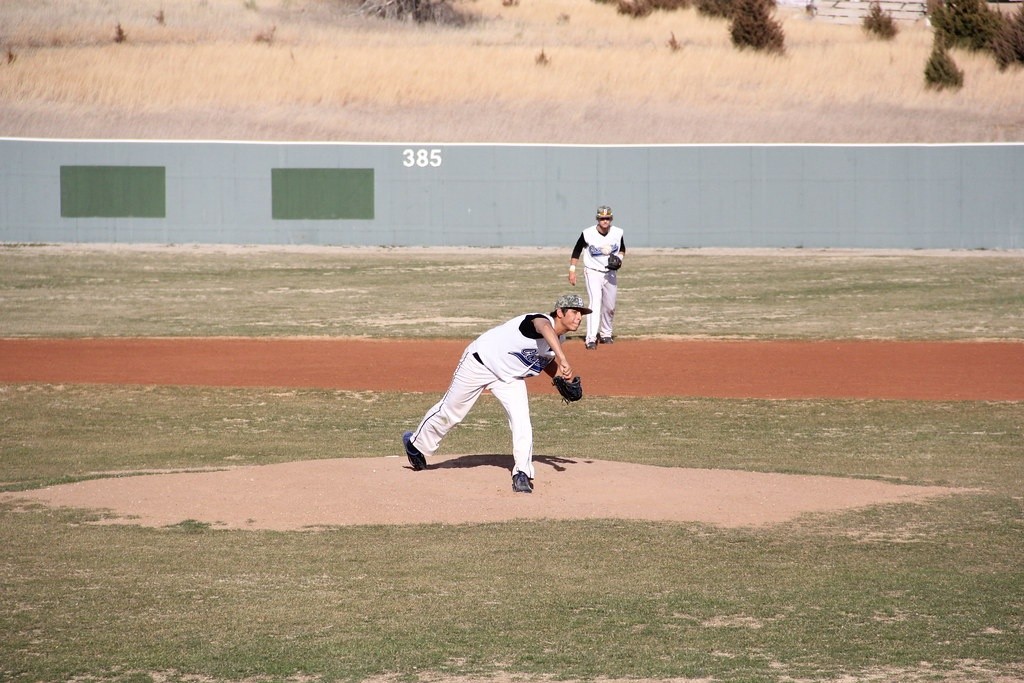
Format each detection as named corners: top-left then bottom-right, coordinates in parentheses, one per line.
top-left (586, 342), bottom-right (596, 349)
top-left (513, 467), bottom-right (532, 492)
top-left (402, 431), bottom-right (427, 470)
top-left (600, 336), bottom-right (613, 344)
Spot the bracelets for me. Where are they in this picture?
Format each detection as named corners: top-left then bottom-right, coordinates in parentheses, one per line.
top-left (569, 265), bottom-right (575, 272)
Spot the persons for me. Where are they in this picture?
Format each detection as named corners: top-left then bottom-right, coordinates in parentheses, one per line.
top-left (403, 294), bottom-right (593, 493)
top-left (568, 205), bottom-right (625, 350)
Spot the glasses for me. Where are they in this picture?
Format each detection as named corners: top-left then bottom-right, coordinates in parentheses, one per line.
top-left (599, 217), bottom-right (611, 220)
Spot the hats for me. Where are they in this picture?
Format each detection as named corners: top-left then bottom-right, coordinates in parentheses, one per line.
top-left (554, 294), bottom-right (593, 315)
top-left (597, 206), bottom-right (613, 217)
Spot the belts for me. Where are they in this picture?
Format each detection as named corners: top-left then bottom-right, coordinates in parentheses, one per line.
top-left (472, 352), bottom-right (484, 365)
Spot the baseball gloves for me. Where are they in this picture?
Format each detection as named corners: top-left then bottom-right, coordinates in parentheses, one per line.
top-left (551, 375), bottom-right (583, 405)
top-left (605, 253), bottom-right (622, 272)
